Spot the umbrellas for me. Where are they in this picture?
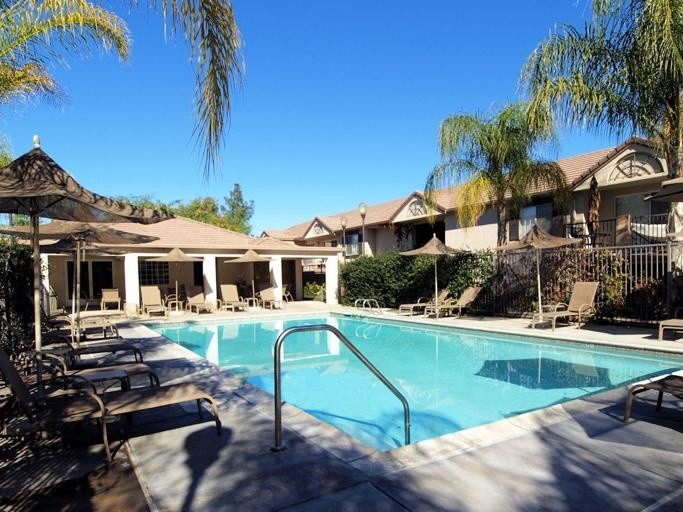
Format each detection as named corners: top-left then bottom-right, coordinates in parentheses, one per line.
top-left (0, 211), bottom-right (162, 361)
top-left (488, 216), bottom-right (584, 320)
top-left (396, 233), bottom-right (471, 319)
top-left (14, 230), bottom-right (128, 342)
top-left (471, 342), bottom-right (613, 393)
top-left (223, 248), bottom-right (276, 307)
top-left (142, 245), bottom-right (203, 313)
top-left (0, 132), bottom-right (177, 392)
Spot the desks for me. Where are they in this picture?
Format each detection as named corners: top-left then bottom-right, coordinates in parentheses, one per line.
top-left (168, 302), bottom-right (183, 311)
top-left (245, 298), bottom-right (260, 306)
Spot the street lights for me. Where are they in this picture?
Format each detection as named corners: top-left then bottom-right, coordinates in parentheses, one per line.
top-left (340, 215), bottom-right (348, 267)
top-left (358, 201), bottom-right (367, 256)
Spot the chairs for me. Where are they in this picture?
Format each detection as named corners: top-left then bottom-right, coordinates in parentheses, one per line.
top-left (139, 286), bottom-right (167, 316)
top-left (283, 284), bottom-right (293, 300)
top-left (622, 372), bottom-right (683, 424)
top-left (260, 287), bottom-right (284, 310)
top-left (34, 283), bottom-right (145, 364)
top-left (220, 284), bottom-right (246, 312)
top-left (531, 280), bottom-right (683, 346)
top-left (1, 358), bottom-right (220, 461)
top-left (399, 282), bottom-right (486, 320)
top-left (25, 348), bottom-right (160, 396)
top-left (185, 286), bottom-right (213, 315)
